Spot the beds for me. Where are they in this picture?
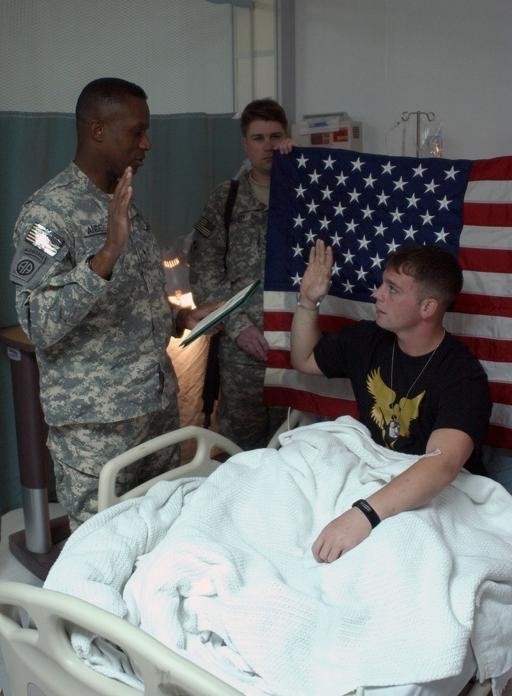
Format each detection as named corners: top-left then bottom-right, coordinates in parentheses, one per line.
top-left (0, 406), bottom-right (510, 696)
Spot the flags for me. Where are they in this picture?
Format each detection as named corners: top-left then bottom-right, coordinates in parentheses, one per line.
top-left (262, 146), bottom-right (512, 450)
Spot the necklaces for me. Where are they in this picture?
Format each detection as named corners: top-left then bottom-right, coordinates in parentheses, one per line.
top-left (388, 327), bottom-right (445, 439)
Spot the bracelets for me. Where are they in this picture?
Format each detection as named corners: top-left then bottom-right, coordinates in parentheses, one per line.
top-left (352, 498), bottom-right (382, 529)
top-left (294, 291), bottom-right (322, 311)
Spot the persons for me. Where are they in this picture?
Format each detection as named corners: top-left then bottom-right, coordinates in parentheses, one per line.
top-left (290, 237), bottom-right (493, 564)
top-left (187, 98), bottom-right (298, 450)
top-left (9, 75), bottom-right (227, 534)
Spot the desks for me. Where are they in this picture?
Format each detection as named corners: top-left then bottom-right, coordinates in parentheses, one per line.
top-left (1, 289), bottom-right (224, 584)
